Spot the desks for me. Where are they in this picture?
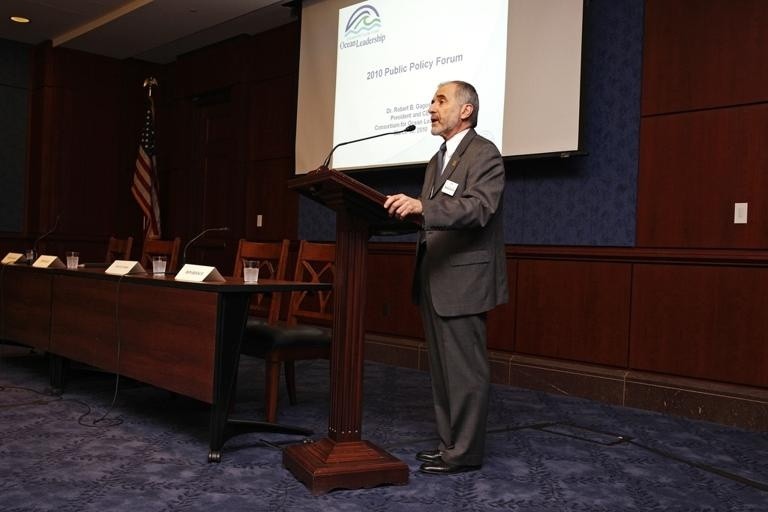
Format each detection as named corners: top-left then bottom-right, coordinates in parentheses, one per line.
top-left (1, 256), bottom-right (330, 464)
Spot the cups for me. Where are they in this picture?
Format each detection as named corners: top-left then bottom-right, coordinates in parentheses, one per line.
top-left (242, 260), bottom-right (259, 283)
top-left (66, 252), bottom-right (79, 270)
top-left (153, 256), bottom-right (168, 275)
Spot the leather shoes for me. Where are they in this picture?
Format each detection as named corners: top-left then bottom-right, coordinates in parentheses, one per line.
top-left (419, 456), bottom-right (481, 475)
top-left (415, 450), bottom-right (445, 463)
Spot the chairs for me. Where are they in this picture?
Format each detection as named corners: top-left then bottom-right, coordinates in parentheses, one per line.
top-left (219, 241), bottom-right (289, 412)
top-left (104, 234), bottom-right (133, 272)
top-left (136, 237), bottom-right (180, 275)
top-left (237, 241), bottom-right (336, 419)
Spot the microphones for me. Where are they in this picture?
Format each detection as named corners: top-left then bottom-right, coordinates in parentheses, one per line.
top-left (305, 124), bottom-right (417, 176)
top-left (174, 226), bottom-right (230, 275)
top-left (32, 215), bottom-right (60, 250)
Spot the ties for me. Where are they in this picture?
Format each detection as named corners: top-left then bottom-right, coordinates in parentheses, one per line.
top-left (432, 141), bottom-right (446, 189)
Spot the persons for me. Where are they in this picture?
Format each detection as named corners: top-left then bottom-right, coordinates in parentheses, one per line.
top-left (383, 80), bottom-right (512, 477)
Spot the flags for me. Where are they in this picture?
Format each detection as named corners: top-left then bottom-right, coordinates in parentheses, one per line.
top-left (129, 102), bottom-right (164, 238)
